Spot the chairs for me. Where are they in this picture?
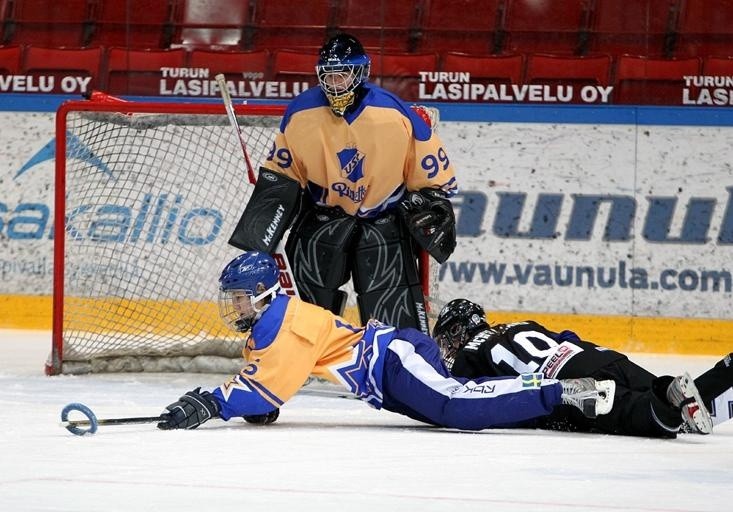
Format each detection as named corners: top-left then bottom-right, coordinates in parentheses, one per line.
top-left (0, 1), bottom-right (732, 107)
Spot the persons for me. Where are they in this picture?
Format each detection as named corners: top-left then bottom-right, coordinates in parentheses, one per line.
top-left (157, 251), bottom-right (596, 430)
top-left (228, 34), bottom-right (458, 337)
top-left (432, 299), bottom-right (733, 439)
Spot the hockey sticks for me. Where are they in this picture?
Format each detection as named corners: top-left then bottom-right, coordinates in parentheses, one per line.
top-left (216, 72), bottom-right (352, 395)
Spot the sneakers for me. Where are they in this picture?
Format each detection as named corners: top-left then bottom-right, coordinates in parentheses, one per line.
top-left (560, 378), bottom-right (596, 419)
top-left (667, 376), bottom-right (713, 434)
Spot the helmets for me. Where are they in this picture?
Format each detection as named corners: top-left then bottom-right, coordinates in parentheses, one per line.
top-left (314, 34), bottom-right (371, 115)
top-left (433, 299), bottom-right (491, 371)
top-left (218, 251), bottom-right (282, 332)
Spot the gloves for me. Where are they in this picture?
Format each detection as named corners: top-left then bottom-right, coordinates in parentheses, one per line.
top-left (158, 387), bottom-right (221, 429)
